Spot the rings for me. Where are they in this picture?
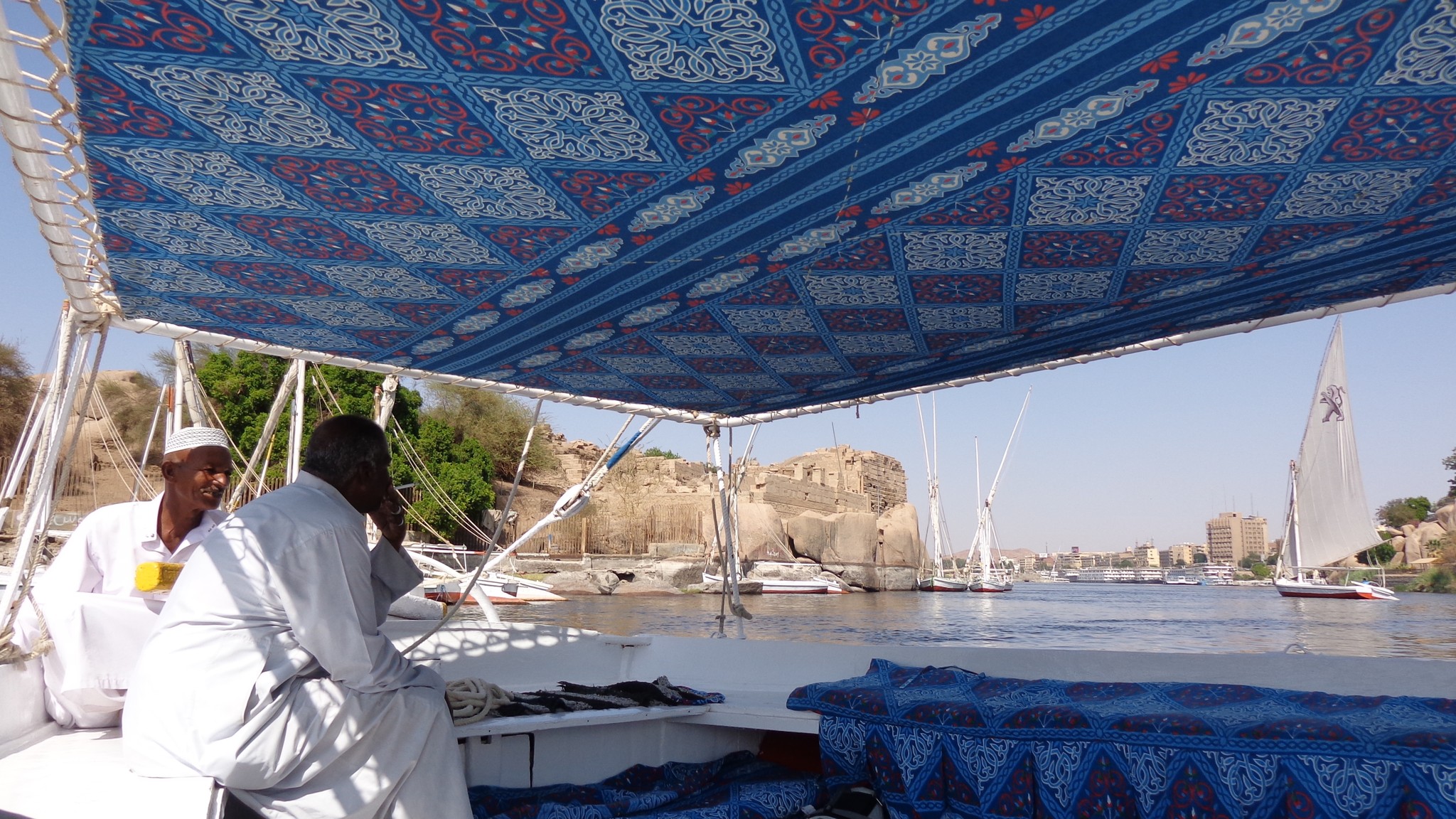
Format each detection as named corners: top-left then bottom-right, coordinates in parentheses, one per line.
top-left (391, 505), bottom-right (402, 514)
top-left (394, 517), bottom-right (404, 526)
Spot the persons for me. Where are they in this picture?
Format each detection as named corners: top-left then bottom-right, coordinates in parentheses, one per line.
top-left (121, 413), bottom-right (474, 819)
top-left (1362, 578), bottom-right (1369, 585)
top-left (10, 427), bottom-right (234, 728)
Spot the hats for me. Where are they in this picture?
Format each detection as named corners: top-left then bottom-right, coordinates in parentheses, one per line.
top-left (164, 426), bottom-right (229, 457)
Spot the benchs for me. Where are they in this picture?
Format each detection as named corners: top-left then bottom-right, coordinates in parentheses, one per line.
top-left (459, 659), bottom-right (1455, 816)
top-left (0, 723), bottom-right (224, 819)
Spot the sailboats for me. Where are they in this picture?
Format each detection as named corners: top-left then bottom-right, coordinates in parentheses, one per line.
top-left (356, 382), bottom-right (571, 605)
top-left (971, 386), bottom-right (1031, 592)
top-left (916, 390), bottom-right (968, 592)
top-left (1271, 313), bottom-right (1399, 602)
top-left (700, 421), bottom-right (850, 594)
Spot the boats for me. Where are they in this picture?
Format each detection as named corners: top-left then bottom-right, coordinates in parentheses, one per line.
top-left (1205, 579), bottom-right (1237, 586)
top-left (1234, 580), bottom-right (1275, 586)
top-left (1162, 576), bottom-right (1198, 585)
